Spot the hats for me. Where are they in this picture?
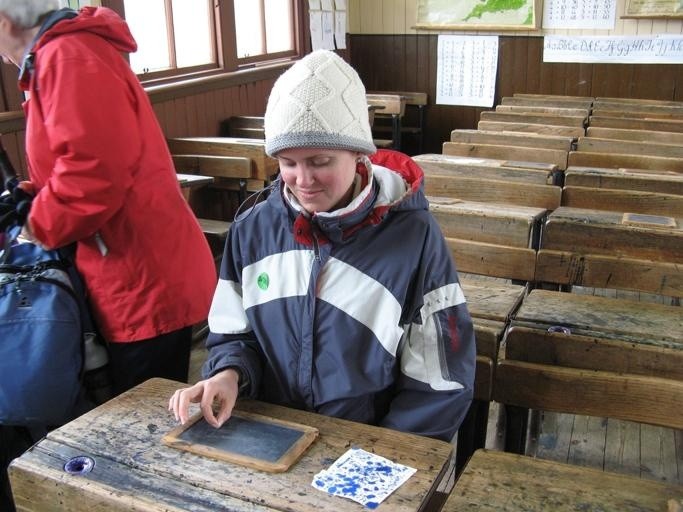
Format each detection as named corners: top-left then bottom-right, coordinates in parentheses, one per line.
top-left (264, 48), bottom-right (377, 159)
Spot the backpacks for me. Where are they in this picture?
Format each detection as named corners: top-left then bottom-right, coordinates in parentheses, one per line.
top-left (1, 181), bottom-right (115, 429)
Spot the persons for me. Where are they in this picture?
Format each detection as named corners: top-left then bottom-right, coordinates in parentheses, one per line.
top-left (166, 49), bottom-right (478, 441)
top-left (1, 1), bottom-right (218, 400)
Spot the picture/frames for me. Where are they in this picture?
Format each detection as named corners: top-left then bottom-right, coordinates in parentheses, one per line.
top-left (621, 0), bottom-right (683, 20)
top-left (411, 0), bottom-right (542, 33)
